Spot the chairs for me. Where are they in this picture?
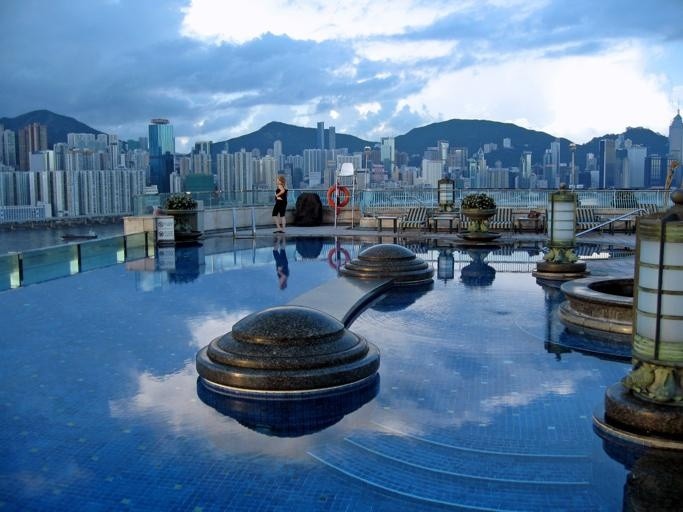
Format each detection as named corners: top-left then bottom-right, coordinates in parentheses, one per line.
top-left (399, 208), bottom-right (429, 231)
top-left (637, 203), bottom-right (658, 217)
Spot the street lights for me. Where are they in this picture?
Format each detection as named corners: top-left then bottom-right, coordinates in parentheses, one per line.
top-left (569, 142), bottom-right (576, 192)
top-left (362, 143), bottom-right (369, 188)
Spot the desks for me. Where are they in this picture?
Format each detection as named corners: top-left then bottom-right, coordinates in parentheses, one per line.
top-left (428, 217), bottom-right (455, 233)
top-left (377, 216), bottom-right (398, 233)
top-left (517, 217), bottom-right (540, 234)
top-left (608, 217), bottom-right (632, 236)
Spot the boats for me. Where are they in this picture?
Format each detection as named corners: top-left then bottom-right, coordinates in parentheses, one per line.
top-left (59, 223), bottom-right (97, 239)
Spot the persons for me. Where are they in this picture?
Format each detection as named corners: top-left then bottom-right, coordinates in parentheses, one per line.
top-left (273, 237), bottom-right (289, 290)
top-left (272, 176), bottom-right (289, 233)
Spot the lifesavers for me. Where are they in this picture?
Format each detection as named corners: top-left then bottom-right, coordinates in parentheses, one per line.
top-left (328, 246), bottom-right (351, 270)
top-left (327, 183), bottom-right (350, 207)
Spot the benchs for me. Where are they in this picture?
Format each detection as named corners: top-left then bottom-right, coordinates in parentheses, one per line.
top-left (457, 206), bottom-right (514, 233)
top-left (543, 208), bottom-right (602, 235)
